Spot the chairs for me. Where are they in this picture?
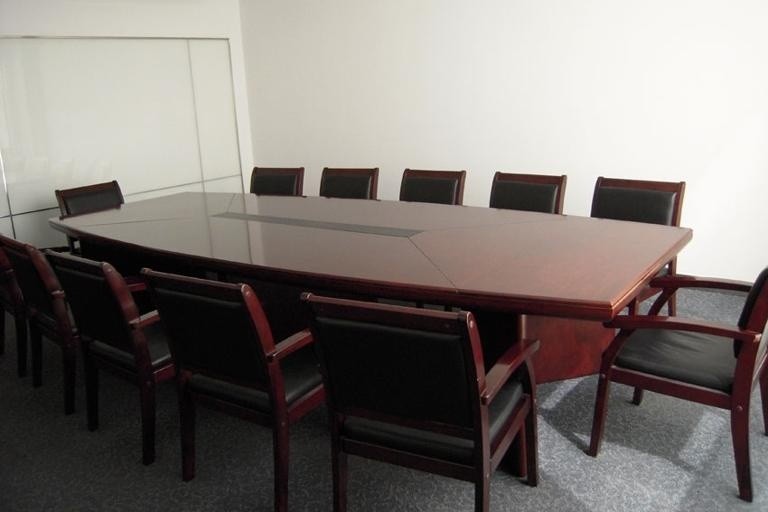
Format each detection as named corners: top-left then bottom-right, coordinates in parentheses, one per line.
top-left (489, 170), bottom-right (567, 215)
top-left (139, 266), bottom-right (330, 510)
top-left (1, 233), bottom-right (31, 378)
top-left (3, 235), bottom-right (84, 417)
top-left (319, 166), bottom-right (380, 201)
top-left (250, 167), bottom-right (305, 197)
top-left (54, 180), bottom-right (127, 255)
top-left (584, 264), bottom-right (767, 503)
top-left (296, 289), bottom-right (545, 511)
top-left (591, 176), bottom-right (686, 317)
top-left (46, 249), bottom-right (179, 468)
top-left (398, 169), bottom-right (467, 205)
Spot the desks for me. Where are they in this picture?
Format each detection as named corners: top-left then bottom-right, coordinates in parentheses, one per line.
top-left (49, 190), bottom-right (694, 490)
